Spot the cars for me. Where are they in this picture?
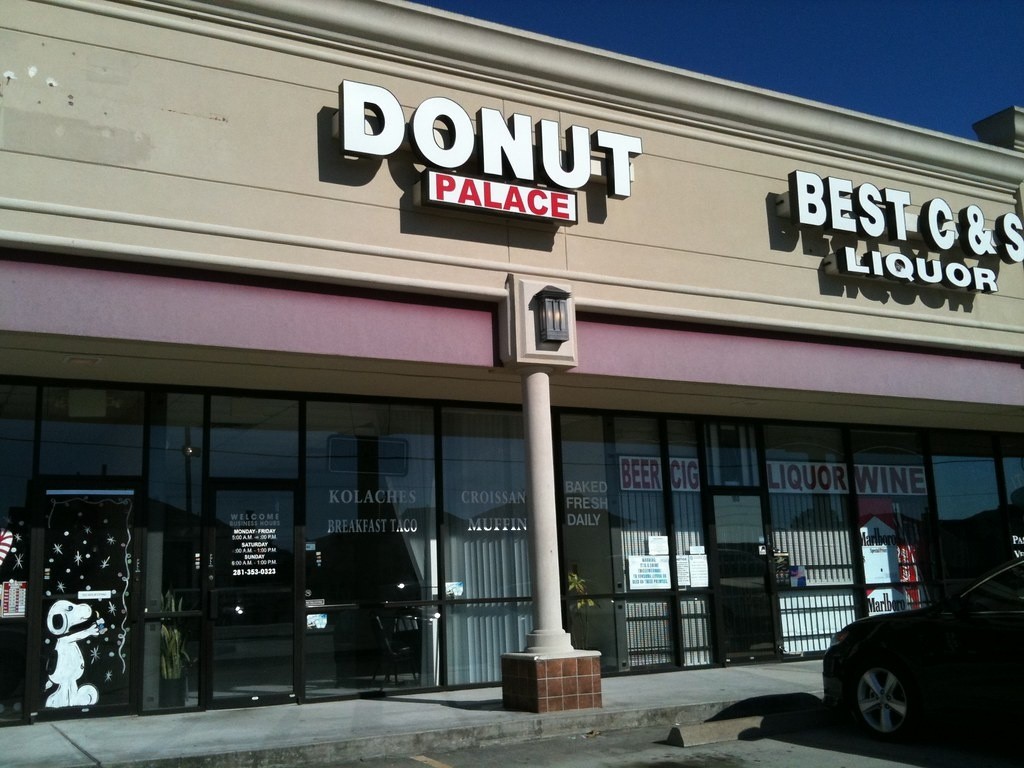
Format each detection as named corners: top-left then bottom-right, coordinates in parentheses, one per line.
top-left (720, 547), bottom-right (770, 643)
top-left (821, 554), bottom-right (1023, 746)
